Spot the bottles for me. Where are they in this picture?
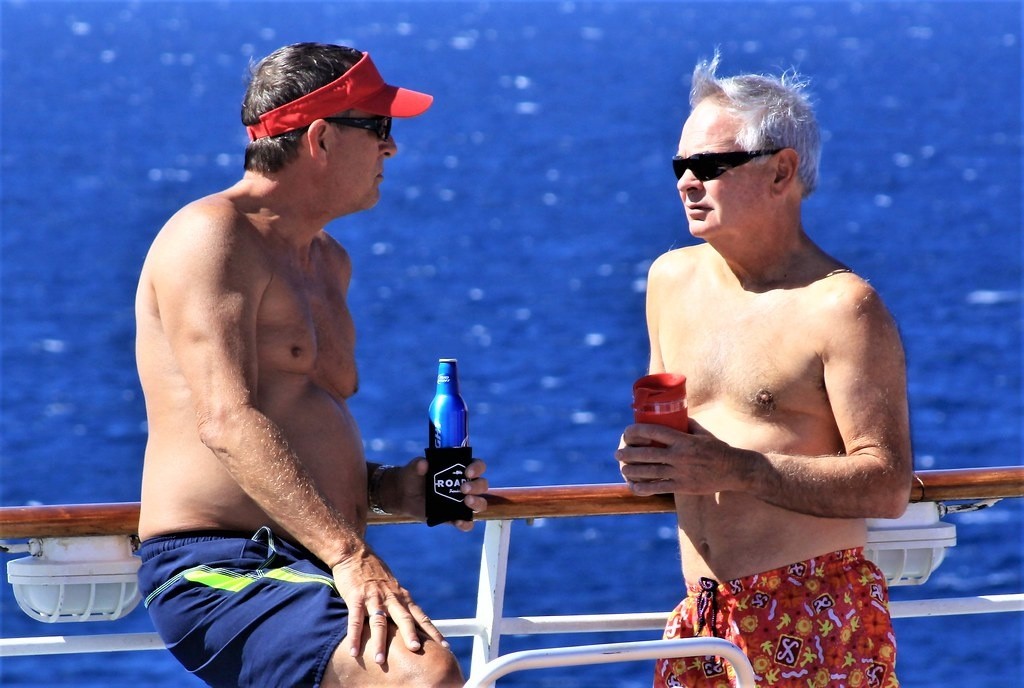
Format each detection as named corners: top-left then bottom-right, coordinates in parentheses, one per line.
top-left (423, 359), bottom-right (469, 449)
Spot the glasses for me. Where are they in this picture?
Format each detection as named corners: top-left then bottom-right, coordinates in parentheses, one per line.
top-left (671, 147), bottom-right (793, 181)
top-left (297, 113), bottom-right (392, 143)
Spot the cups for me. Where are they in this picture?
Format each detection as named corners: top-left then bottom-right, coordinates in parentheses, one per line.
top-left (630, 374), bottom-right (690, 476)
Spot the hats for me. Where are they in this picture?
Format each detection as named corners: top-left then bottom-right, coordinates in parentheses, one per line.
top-left (245, 48), bottom-right (437, 143)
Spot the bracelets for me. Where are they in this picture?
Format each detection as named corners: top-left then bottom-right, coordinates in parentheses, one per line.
top-left (367, 462), bottom-right (398, 517)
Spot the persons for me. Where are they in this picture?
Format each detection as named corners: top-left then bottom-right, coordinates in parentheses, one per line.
top-left (128, 36), bottom-right (473, 688)
top-left (608, 42), bottom-right (918, 688)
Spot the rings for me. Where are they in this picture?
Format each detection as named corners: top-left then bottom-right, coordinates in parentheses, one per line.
top-left (627, 480), bottom-right (638, 491)
top-left (368, 610), bottom-right (388, 619)
